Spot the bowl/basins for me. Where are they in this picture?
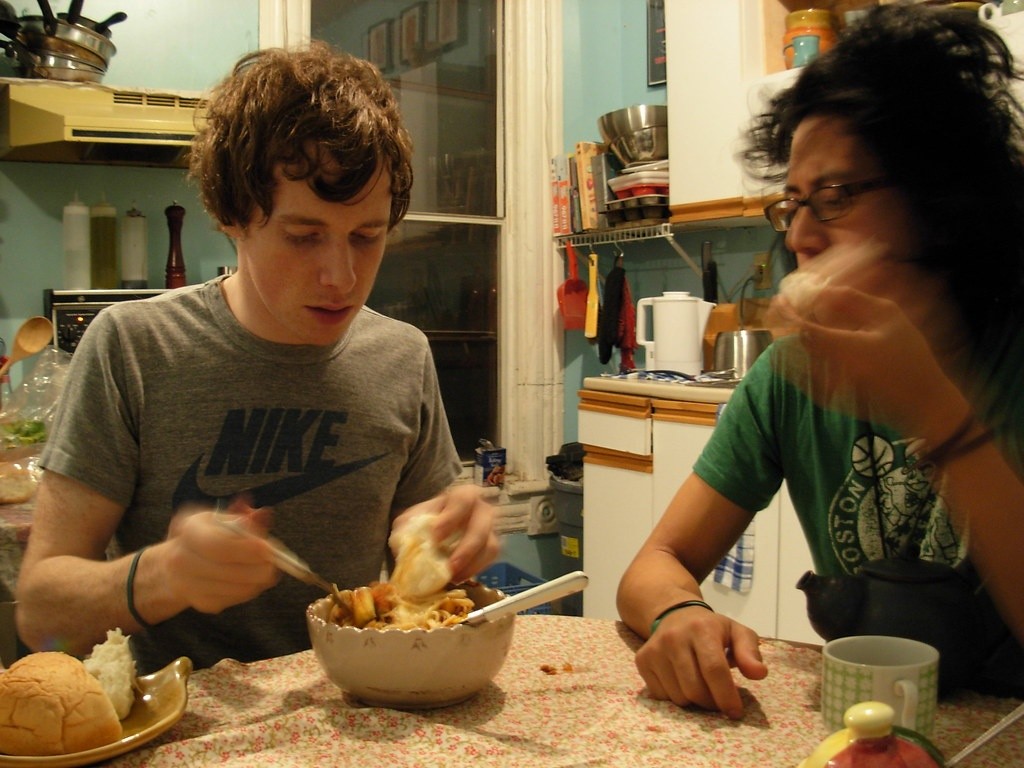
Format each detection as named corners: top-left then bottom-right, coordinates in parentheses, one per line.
top-left (305, 576), bottom-right (516, 711)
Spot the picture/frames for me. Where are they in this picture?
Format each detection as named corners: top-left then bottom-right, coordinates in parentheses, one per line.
top-left (366, 0), bottom-right (469, 72)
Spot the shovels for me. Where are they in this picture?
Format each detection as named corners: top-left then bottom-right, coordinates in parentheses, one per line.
top-left (558, 245), bottom-right (584, 330)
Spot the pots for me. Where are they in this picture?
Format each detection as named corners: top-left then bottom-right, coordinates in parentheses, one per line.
top-left (706, 330), bottom-right (774, 380)
top-left (0, 0), bottom-right (127, 84)
top-left (597, 105), bottom-right (668, 168)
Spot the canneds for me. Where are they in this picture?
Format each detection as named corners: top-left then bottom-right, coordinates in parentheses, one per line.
top-left (474, 439), bottom-right (506, 487)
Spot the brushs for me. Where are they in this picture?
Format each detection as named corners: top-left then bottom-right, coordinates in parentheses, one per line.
top-left (584, 249), bottom-right (602, 342)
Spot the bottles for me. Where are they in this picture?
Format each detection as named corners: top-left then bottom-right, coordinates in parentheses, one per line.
top-left (61, 188), bottom-right (92, 290)
top-left (90, 190), bottom-right (117, 290)
top-left (0, 356), bottom-right (13, 414)
top-left (782, 8), bottom-right (842, 70)
top-left (120, 200), bottom-right (148, 290)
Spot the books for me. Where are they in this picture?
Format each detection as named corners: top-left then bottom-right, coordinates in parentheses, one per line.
top-left (549, 141), bottom-right (622, 238)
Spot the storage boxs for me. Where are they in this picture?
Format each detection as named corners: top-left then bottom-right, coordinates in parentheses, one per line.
top-left (476, 560), bottom-right (556, 615)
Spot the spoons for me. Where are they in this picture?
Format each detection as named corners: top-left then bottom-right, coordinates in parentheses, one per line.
top-left (0, 316), bottom-right (53, 378)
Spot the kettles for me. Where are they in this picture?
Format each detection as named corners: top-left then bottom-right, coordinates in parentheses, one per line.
top-left (635, 291), bottom-right (717, 380)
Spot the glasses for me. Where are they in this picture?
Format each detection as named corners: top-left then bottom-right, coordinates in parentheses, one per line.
top-left (765, 175), bottom-right (891, 232)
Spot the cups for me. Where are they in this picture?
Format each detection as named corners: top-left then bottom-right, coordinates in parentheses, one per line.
top-left (821, 634), bottom-right (940, 746)
top-left (978, 0), bottom-right (1024, 22)
top-left (783, 35), bottom-right (820, 69)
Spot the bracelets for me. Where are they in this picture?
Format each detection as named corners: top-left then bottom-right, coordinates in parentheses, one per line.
top-left (650, 599), bottom-right (714, 635)
top-left (915, 412), bottom-right (996, 469)
top-left (127, 547), bottom-right (152, 628)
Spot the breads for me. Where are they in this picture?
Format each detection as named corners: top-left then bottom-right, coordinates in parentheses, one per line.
top-left (81, 628), bottom-right (137, 719)
top-left (0, 652), bottom-right (123, 756)
top-left (388, 513), bottom-right (451, 596)
top-left (0, 464), bottom-right (40, 504)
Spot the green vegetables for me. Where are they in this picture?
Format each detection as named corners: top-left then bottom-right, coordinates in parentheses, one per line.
top-left (0, 420), bottom-right (47, 448)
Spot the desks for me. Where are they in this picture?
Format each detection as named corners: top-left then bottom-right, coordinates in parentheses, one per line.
top-left (0, 612), bottom-right (1024, 768)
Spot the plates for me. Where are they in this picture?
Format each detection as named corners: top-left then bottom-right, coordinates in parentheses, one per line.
top-left (0, 656), bottom-right (192, 768)
top-left (607, 160), bottom-right (670, 200)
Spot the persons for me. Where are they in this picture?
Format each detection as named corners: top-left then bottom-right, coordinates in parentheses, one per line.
top-left (15, 44), bottom-right (499, 680)
top-left (616, 0), bottom-right (1024, 721)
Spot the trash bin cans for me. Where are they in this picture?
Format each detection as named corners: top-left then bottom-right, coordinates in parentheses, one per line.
top-left (551, 476), bottom-right (583, 617)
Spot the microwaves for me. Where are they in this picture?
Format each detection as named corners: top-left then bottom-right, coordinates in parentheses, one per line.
top-left (43, 288), bottom-right (172, 366)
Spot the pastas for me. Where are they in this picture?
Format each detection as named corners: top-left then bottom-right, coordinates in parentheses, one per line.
top-left (328, 588), bottom-right (475, 631)
